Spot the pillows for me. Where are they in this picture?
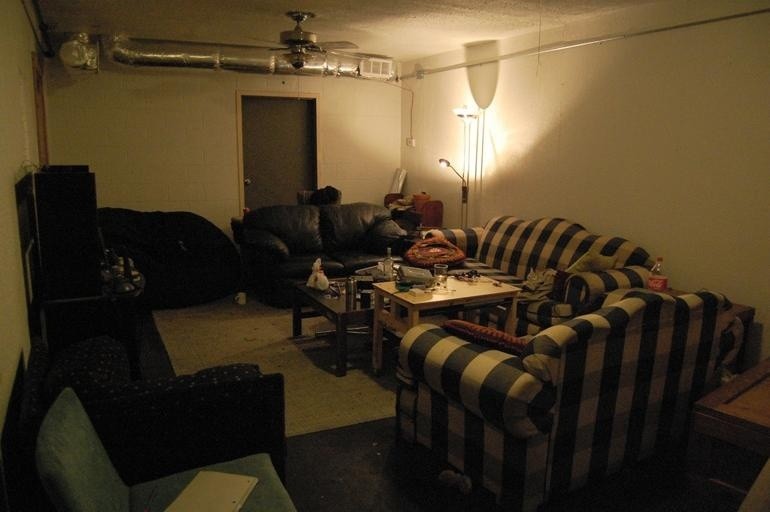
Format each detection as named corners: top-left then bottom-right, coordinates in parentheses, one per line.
top-left (438, 316), bottom-right (527, 356)
top-left (403, 237), bottom-right (466, 270)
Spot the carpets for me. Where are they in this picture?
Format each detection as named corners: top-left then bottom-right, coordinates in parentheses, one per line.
top-left (150, 291), bottom-right (399, 438)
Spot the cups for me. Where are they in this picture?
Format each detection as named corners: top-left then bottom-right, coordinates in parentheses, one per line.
top-left (434, 264), bottom-right (449, 277)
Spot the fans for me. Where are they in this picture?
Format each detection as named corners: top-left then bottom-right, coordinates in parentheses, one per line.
top-left (243, 10), bottom-right (370, 60)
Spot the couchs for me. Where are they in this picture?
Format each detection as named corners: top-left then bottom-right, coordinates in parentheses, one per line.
top-left (97, 206), bottom-right (242, 311)
top-left (422, 216), bottom-right (660, 338)
top-left (239, 202), bottom-right (409, 310)
top-left (394, 287), bottom-right (746, 512)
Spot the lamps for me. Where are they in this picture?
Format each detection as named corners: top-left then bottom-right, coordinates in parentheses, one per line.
top-left (282, 47), bottom-right (313, 70)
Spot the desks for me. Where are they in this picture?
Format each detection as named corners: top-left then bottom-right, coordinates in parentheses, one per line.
top-left (42, 249), bottom-right (147, 380)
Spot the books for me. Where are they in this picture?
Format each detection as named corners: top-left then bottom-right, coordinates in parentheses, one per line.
top-left (163, 471), bottom-right (258, 512)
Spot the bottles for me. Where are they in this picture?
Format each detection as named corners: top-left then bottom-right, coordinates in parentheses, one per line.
top-left (648, 256), bottom-right (668, 293)
top-left (345, 276), bottom-right (357, 295)
top-left (384, 248), bottom-right (394, 281)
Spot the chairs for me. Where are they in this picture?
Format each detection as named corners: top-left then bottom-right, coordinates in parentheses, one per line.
top-left (18, 336), bottom-right (297, 512)
